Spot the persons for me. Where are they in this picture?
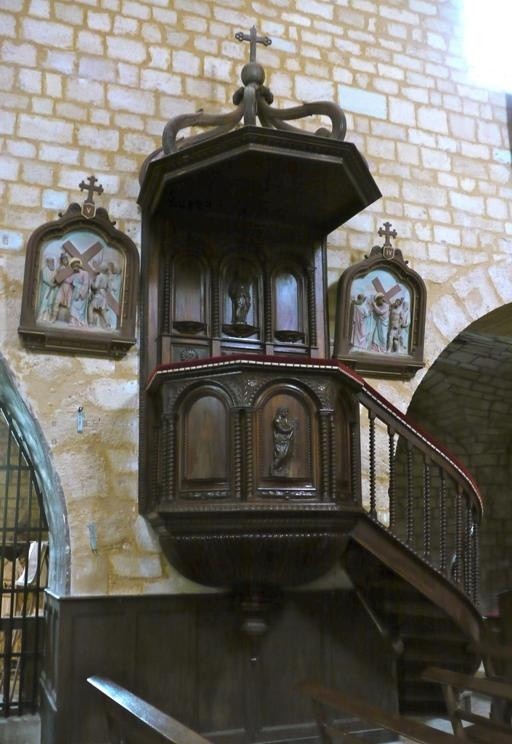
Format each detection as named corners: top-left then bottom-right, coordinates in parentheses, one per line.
top-left (371, 291), bottom-right (389, 352)
top-left (385, 297), bottom-right (403, 353)
top-left (228, 267), bottom-right (251, 325)
top-left (36, 252), bottom-right (120, 329)
top-left (352, 293), bottom-right (371, 351)
top-left (269, 404), bottom-right (300, 476)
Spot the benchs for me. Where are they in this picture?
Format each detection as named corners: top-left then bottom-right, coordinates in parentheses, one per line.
top-left (84, 638), bottom-right (510, 743)
